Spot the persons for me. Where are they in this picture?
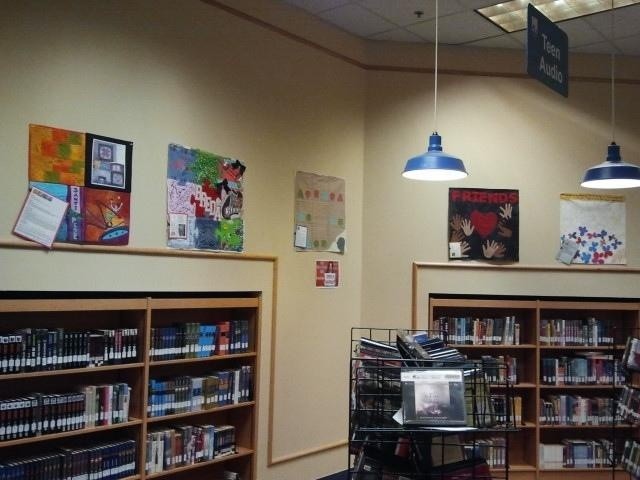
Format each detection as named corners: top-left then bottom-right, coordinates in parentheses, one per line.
top-left (322, 261), bottom-right (337, 287)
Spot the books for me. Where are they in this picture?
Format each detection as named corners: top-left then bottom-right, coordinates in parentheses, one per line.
top-left (538, 392), bottom-right (619, 469)
top-left (467, 394), bottom-right (521, 469)
top-left (1, 320), bottom-right (139, 479)
top-left (538, 316), bottom-right (627, 387)
top-left (352, 331), bottom-right (490, 479)
top-left (145, 321), bottom-right (251, 479)
top-left (616, 336), bottom-right (640, 479)
top-left (431, 316), bottom-right (522, 385)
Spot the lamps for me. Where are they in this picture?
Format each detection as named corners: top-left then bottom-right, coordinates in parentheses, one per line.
top-left (402, 0), bottom-right (469, 182)
top-left (580, 0), bottom-right (640, 189)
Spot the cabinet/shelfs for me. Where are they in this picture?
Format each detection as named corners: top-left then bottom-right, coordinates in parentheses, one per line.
top-left (428, 293), bottom-right (640, 480)
top-left (611, 326), bottom-right (640, 480)
top-left (350, 327), bottom-right (509, 480)
top-left (0, 288), bottom-right (263, 480)
